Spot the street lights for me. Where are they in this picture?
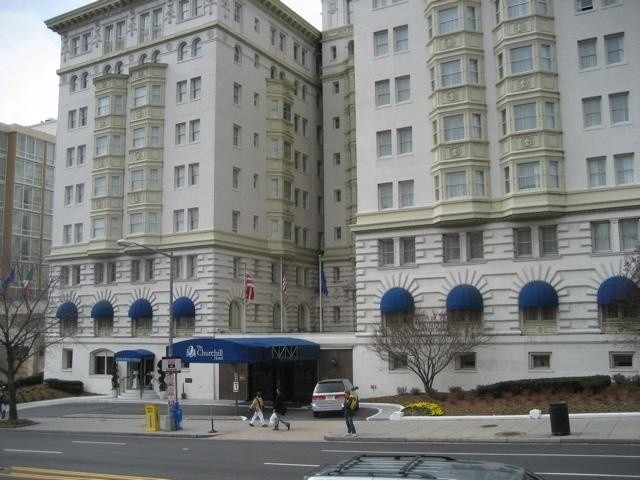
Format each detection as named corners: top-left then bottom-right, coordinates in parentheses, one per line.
top-left (116, 238), bottom-right (175, 431)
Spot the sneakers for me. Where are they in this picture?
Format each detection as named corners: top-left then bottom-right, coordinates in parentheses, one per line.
top-left (250, 424), bottom-right (255, 426)
top-left (288, 423), bottom-right (290, 430)
top-left (353, 434), bottom-right (357, 437)
top-left (262, 424), bottom-right (268, 427)
top-left (344, 433), bottom-right (352, 436)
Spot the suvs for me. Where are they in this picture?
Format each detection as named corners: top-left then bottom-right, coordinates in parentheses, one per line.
top-left (298, 450), bottom-right (543, 480)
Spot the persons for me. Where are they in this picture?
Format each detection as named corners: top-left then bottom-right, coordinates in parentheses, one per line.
top-left (248, 391), bottom-right (268, 428)
top-left (272, 387), bottom-right (290, 431)
top-left (342, 390), bottom-right (357, 437)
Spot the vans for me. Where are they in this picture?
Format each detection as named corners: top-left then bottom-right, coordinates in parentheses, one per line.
top-left (310, 378), bottom-right (360, 418)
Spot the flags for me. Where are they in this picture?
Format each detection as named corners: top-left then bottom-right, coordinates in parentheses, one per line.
top-left (0, 267), bottom-right (15, 295)
top-left (20, 267), bottom-right (33, 296)
top-left (282, 262), bottom-right (289, 295)
top-left (245, 269), bottom-right (255, 301)
top-left (320, 260), bottom-right (328, 296)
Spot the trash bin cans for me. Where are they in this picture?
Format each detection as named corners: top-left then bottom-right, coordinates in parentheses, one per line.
top-left (549, 401), bottom-right (570, 436)
